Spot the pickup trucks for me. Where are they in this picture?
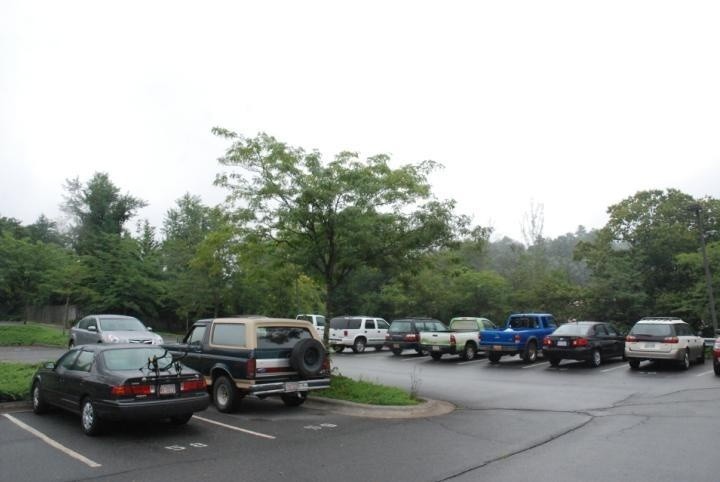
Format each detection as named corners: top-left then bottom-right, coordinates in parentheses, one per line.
top-left (418, 317), bottom-right (498, 360)
top-left (478, 313), bottom-right (558, 363)
top-left (295, 314), bottom-right (325, 339)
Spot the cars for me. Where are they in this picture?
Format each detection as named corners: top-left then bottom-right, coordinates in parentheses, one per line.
top-left (543, 320), bottom-right (625, 368)
top-left (30, 344), bottom-right (210, 436)
top-left (68, 314), bottom-right (164, 349)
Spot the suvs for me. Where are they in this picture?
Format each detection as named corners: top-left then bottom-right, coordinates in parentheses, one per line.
top-left (159, 315), bottom-right (331, 412)
top-left (329, 315), bottom-right (390, 353)
top-left (624, 316), bottom-right (705, 369)
top-left (384, 317), bottom-right (448, 357)
